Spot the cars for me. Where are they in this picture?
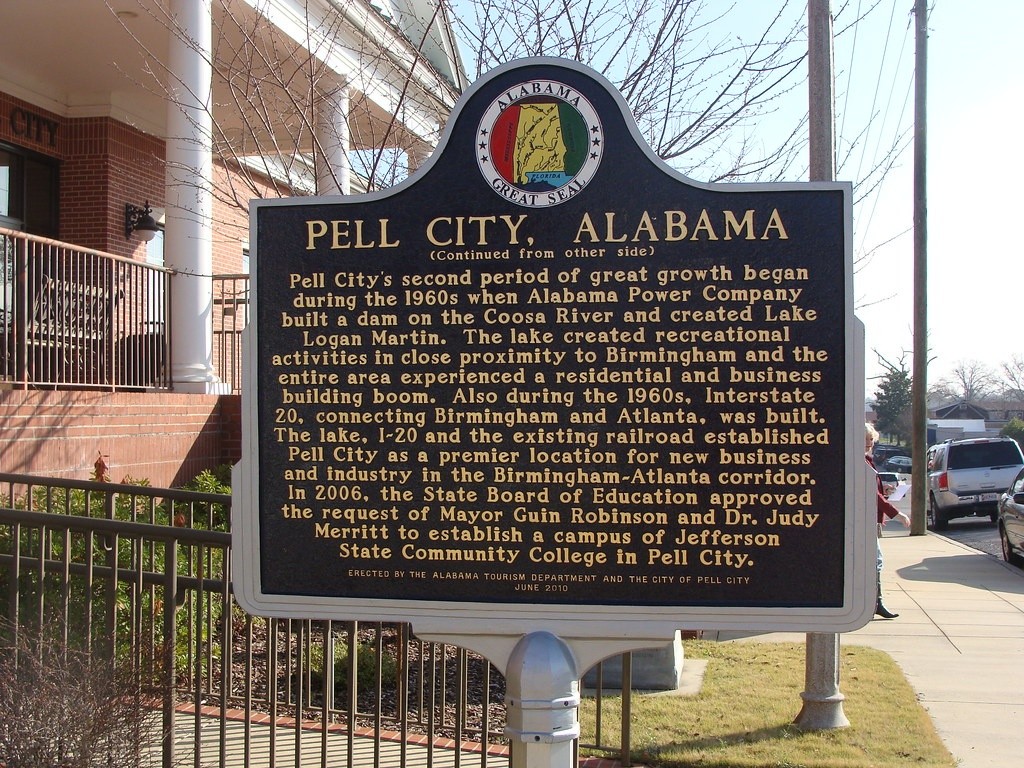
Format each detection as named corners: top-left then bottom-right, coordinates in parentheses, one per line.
top-left (883, 456), bottom-right (912, 474)
top-left (873, 444), bottom-right (906, 465)
top-left (996, 465), bottom-right (1024, 570)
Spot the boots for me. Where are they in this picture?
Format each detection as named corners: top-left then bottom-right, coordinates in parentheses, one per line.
top-left (875, 573), bottom-right (899, 618)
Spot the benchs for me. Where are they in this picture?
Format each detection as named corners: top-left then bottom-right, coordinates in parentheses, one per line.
top-left (0, 273), bottom-right (125, 390)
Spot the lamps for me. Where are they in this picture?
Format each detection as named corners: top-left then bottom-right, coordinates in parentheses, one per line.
top-left (125, 200), bottom-right (158, 244)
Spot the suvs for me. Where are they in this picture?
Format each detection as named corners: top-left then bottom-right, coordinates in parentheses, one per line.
top-left (878, 471), bottom-right (908, 499)
top-left (927, 434), bottom-right (1024, 532)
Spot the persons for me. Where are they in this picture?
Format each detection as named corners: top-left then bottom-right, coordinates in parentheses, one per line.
top-left (865, 422), bottom-right (911, 619)
